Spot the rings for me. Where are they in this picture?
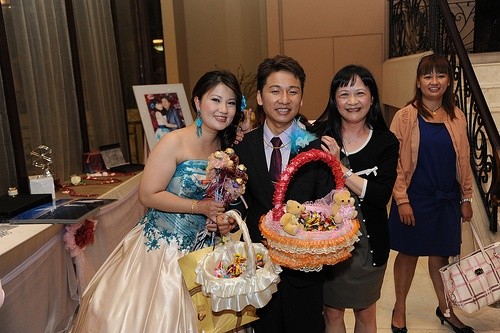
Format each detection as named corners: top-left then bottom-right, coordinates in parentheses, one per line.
top-left (218, 212), bottom-right (221, 216)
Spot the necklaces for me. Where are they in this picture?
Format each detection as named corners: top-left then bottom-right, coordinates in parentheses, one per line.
top-left (63, 188), bottom-right (99, 196)
top-left (264, 140), bottom-right (293, 148)
top-left (81, 178), bottom-right (122, 185)
top-left (347, 134), bottom-right (361, 144)
top-left (422, 101), bottom-right (443, 115)
top-left (82, 170), bottom-right (137, 177)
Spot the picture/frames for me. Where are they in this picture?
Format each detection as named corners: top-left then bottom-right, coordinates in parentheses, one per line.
top-left (133, 83), bottom-right (193, 151)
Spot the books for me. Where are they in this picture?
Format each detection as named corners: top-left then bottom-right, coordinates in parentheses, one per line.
top-left (8, 197), bottom-right (118, 223)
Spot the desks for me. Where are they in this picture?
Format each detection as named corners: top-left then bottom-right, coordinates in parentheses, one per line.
top-left (0, 172), bottom-right (151, 333)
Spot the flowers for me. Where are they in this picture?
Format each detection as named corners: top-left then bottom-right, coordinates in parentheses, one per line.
top-left (205, 148), bottom-right (248, 249)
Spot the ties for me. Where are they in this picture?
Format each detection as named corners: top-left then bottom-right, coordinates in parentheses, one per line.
top-left (268, 136), bottom-right (283, 185)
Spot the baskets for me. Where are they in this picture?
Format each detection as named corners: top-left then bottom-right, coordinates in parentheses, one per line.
top-left (195, 210), bottom-right (282, 311)
top-left (258, 149), bottom-right (362, 273)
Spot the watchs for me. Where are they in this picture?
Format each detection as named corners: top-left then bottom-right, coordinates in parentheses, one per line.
top-left (344, 168), bottom-right (352, 179)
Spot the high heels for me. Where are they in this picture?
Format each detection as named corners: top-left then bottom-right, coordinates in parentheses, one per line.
top-left (391, 309), bottom-right (407, 333)
top-left (437, 306), bottom-right (473, 333)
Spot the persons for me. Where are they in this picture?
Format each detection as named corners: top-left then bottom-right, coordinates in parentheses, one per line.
top-left (391, 53), bottom-right (475, 333)
top-left (65, 71), bottom-right (246, 333)
top-left (216, 55), bottom-right (337, 333)
top-left (149, 95), bottom-right (186, 138)
top-left (232, 65), bottom-right (400, 333)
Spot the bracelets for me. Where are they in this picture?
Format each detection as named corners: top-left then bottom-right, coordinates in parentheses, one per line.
top-left (460, 199), bottom-right (472, 203)
top-left (192, 199), bottom-right (195, 214)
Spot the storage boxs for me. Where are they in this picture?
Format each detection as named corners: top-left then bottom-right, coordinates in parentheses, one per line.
top-left (81, 152), bottom-right (105, 174)
top-left (28, 173), bottom-right (55, 200)
top-left (0, 193), bottom-right (52, 220)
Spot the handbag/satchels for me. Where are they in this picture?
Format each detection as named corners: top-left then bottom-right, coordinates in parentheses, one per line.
top-left (438, 216), bottom-right (500, 313)
top-left (178, 231), bottom-right (260, 333)
